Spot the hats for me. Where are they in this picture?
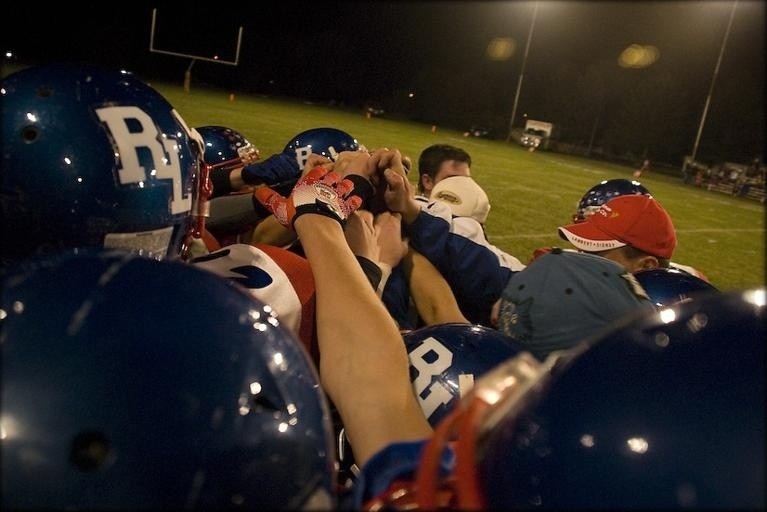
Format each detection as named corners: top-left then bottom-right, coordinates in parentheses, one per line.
top-left (429, 175), bottom-right (492, 224)
top-left (499, 245), bottom-right (658, 355)
top-left (558, 192), bottom-right (677, 260)
top-left (186, 241), bottom-right (316, 370)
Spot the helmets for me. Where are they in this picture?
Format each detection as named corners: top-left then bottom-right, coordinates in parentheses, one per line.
top-left (632, 261), bottom-right (722, 301)
top-left (2, 65), bottom-right (205, 263)
top-left (401, 322), bottom-right (539, 435)
top-left (572, 179), bottom-right (650, 222)
top-left (2, 248), bottom-right (335, 512)
top-left (282, 127), bottom-right (360, 172)
top-left (191, 125), bottom-right (260, 164)
top-left (483, 286), bottom-right (766, 511)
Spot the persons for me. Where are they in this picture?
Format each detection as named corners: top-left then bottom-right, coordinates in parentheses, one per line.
top-left (0, 67), bottom-right (767, 511)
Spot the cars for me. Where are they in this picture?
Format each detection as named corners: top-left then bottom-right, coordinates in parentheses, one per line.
top-left (365, 100), bottom-right (385, 117)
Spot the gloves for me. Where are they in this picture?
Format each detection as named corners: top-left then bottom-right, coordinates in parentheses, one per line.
top-left (255, 165), bottom-right (363, 232)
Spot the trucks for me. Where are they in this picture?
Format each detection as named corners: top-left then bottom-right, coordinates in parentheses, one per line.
top-left (519, 118), bottom-right (557, 154)
